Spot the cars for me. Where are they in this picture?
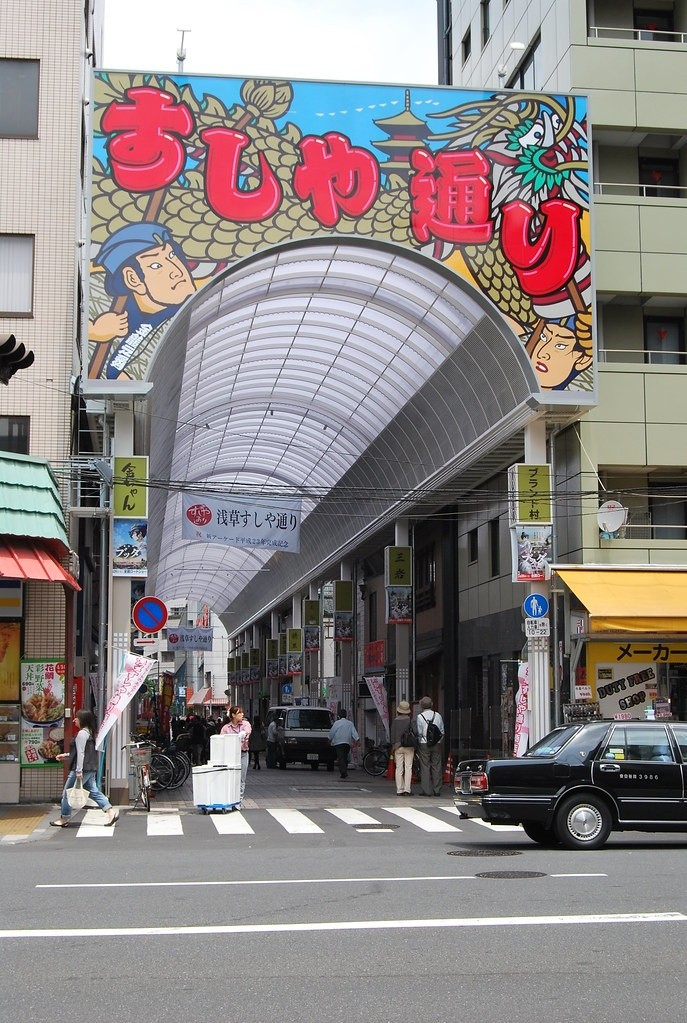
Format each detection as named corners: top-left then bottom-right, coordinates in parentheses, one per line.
top-left (451, 717), bottom-right (687, 851)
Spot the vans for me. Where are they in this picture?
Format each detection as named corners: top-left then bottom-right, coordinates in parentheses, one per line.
top-left (261, 704), bottom-right (338, 772)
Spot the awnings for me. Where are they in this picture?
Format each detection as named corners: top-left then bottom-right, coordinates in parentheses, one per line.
top-left (551, 564), bottom-right (687, 635)
top-left (187, 687), bottom-right (229, 706)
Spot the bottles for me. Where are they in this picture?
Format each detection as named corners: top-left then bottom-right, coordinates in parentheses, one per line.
top-left (563, 701), bottom-right (602, 724)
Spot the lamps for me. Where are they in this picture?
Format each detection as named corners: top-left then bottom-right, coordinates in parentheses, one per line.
top-left (93, 460), bottom-right (114, 487)
top-left (524, 391), bottom-right (597, 413)
top-left (70, 374), bottom-right (155, 400)
top-left (78, 240), bottom-right (85, 248)
top-left (84, 98), bottom-right (90, 106)
top-left (85, 48), bottom-right (93, 60)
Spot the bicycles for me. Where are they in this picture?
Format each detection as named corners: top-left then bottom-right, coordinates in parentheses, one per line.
top-left (121, 731), bottom-right (191, 812)
top-left (363, 743), bottom-right (421, 777)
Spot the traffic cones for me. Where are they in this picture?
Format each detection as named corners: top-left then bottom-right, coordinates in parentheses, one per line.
top-left (385, 751), bottom-right (395, 780)
top-left (444, 751), bottom-right (454, 783)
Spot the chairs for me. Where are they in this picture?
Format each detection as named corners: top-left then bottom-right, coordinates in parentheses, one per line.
top-left (650, 745), bottom-right (674, 762)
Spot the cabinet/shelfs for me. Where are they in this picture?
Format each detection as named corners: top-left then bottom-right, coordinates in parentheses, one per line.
top-left (0, 704), bottom-right (20, 806)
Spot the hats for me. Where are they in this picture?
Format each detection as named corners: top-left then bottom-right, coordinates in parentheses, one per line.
top-left (419, 696), bottom-right (433, 708)
top-left (397, 701), bottom-right (412, 714)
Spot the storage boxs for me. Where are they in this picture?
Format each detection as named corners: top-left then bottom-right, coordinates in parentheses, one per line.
top-left (7, 734), bottom-right (16, 741)
top-left (6, 754), bottom-right (15, 761)
top-left (0, 716), bottom-right (7, 722)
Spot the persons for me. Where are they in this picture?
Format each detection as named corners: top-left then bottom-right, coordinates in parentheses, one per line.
top-left (670, 692), bottom-right (681, 720)
top-left (391, 701), bottom-right (417, 796)
top-left (417, 696), bottom-right (444, 796)
top-left (220, 706), bottom-right (252, 811)
top-left (169, 714), bottom-right (232, 765)
top-left (328, 708), bottom-right (361, 778)
top-left (243, 715), bottom-right (282, 770)
top-left (49, 708), bottom-right (119, 828)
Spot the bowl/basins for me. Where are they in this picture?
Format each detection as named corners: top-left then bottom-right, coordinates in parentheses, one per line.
top-left (38, 747), bottom-right (61, 763)
top-left (49, 727), bottom-right (64, 745)
top-left (22, 706), bottom-right (64, 728)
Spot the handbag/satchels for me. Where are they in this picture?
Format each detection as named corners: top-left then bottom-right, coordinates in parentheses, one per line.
top-left (401, 716), bottom-right (417, 746)
top-left (261, 725), bottom-right (265, 736)
top-left (66, 777), bottom-right (90, 810)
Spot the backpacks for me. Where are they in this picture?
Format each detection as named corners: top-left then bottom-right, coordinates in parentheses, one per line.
top-left (420, 710), bottom-right (442, 747)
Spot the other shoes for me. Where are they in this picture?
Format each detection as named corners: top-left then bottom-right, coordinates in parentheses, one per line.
top-left (258, 765), bottom-right (260, 770)
top-left (404, 791), bottom-right (415, 796)
top-left (50, 820), bottom-right (69, 827)
top-left (397, 793), bottom-right (403, 797)
top-left (420, 792), bottom-right (431, 797)
top-left (253, 766), bottom-right (256, 769)
top-left (341, 773), bottom-right (348, 778)
top-left (235, 804), bottom-right (240, 811)
top-left (105, 813), bottom-right (119, 826)
top-left (435, 793), bottom-right (440, 796)
